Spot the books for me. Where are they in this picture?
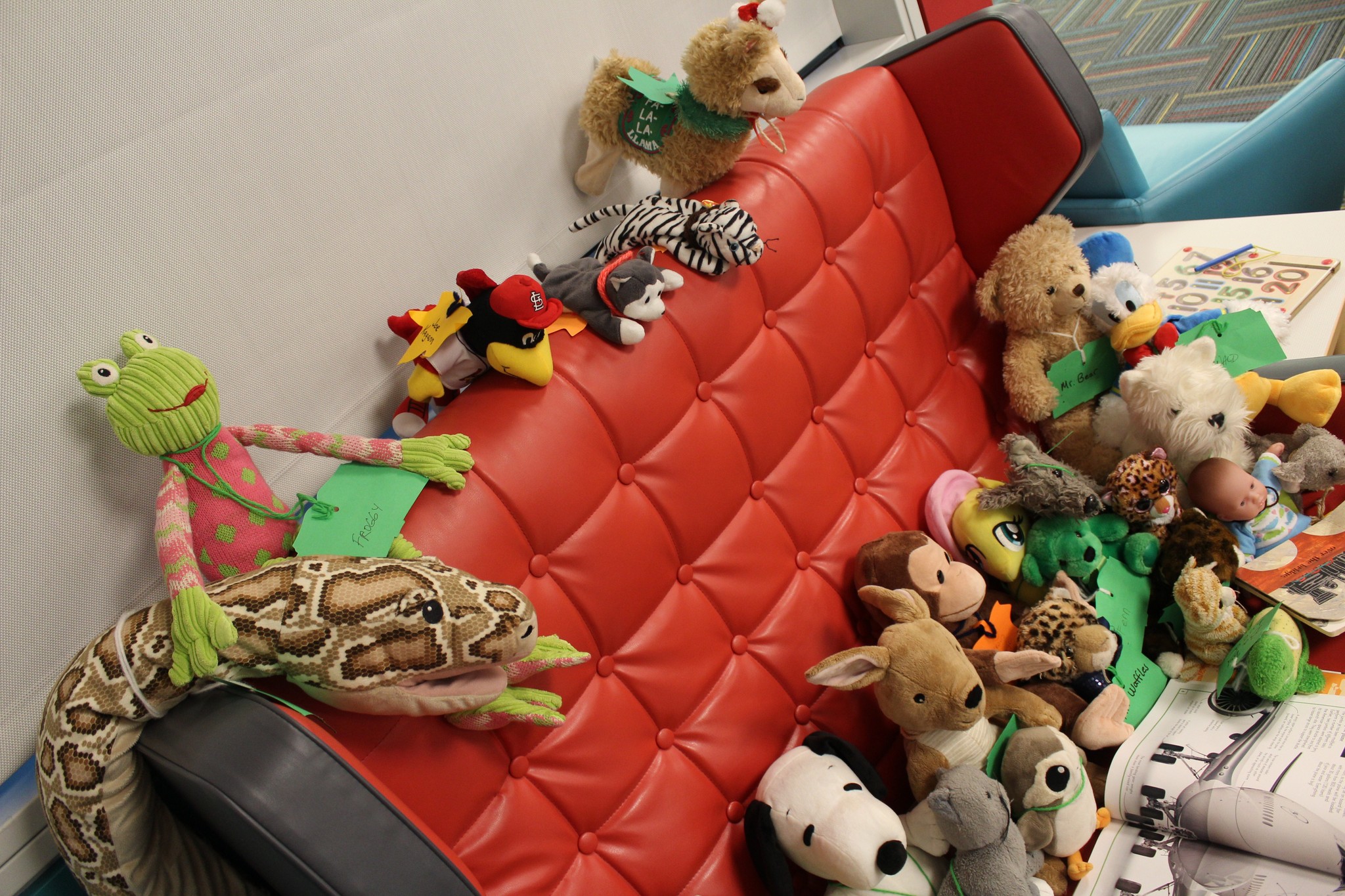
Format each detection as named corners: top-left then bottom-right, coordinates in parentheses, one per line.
top-left (1233, 501), bottom-right (1345, 638)
top-left (1075, 678), bottom-right (1345, 896)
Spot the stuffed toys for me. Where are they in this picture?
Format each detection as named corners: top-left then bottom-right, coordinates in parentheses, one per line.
top-left (567, 194), bottom-right (779, 276)
top-left (525, 245), bottom-right (687, 347)
top-left (805, 214), bottom-right (1345, 896)
top-left (386, 268), bottom-right (564, 438)
top-left (72, 327), bottom-right (592, 731)
top-left (744, 730), bottom-right (1054, 896)
top-left (573, 0), bottom-right (807, 199)
top-left (34, 554), bottom-right (537, 896)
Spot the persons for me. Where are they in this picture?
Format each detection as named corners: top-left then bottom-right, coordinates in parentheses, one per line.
top-left (1191, 442), bottom-right (1311, 563)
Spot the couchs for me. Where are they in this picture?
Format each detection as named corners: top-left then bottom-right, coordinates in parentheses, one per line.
top-left (138, 5), bottom-right (1345, 896)
top-left (1050, 59), bottom-right (1345, 228)
